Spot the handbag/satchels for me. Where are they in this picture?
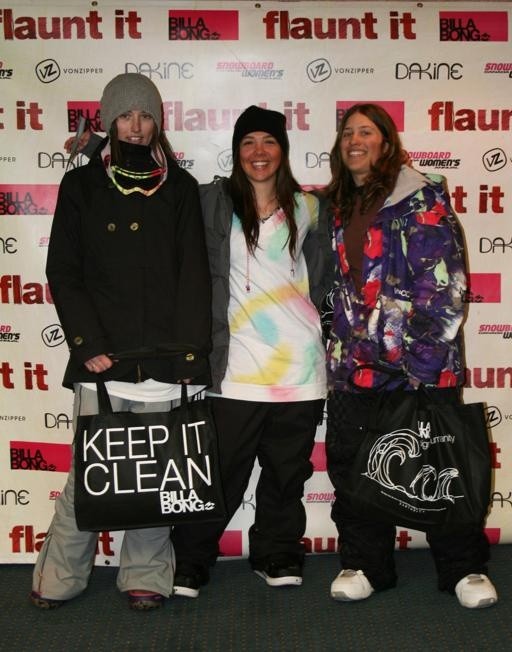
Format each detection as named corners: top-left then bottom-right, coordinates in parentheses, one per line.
top-left (65, 351), bottom-right (231, 534)
top-left (322, 361), bottom-right (500, 532)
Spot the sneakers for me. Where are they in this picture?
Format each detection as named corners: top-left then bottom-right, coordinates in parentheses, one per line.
top-left (253, 555), bottom-right (305, 588)
top-left (330, 564), bottom-right (375, 607)
top-left (126, 590), bottom-right (163, 613)
top-left (28, 588), bottom-right (68, 610)
top-left (176, 568), bottom-right (202, 600)
top-left (453, 573), bottom-right (499, 612)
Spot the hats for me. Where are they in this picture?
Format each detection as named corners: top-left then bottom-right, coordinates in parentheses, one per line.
top-left (229, 106), bottom-right (291, 158)
top-left (99, 72), bottom-right (162, 147)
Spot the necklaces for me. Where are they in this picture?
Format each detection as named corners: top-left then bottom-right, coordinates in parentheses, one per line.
top-left (257, 203), bottom-right (281, 224)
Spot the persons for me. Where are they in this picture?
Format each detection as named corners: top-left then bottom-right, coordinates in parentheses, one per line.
top-left (27, 73), bottom-right (213, 613)
top-left (64, 104), bottom-right (328, 600)
top-left (316, 104), bottom-right (501, 612)
